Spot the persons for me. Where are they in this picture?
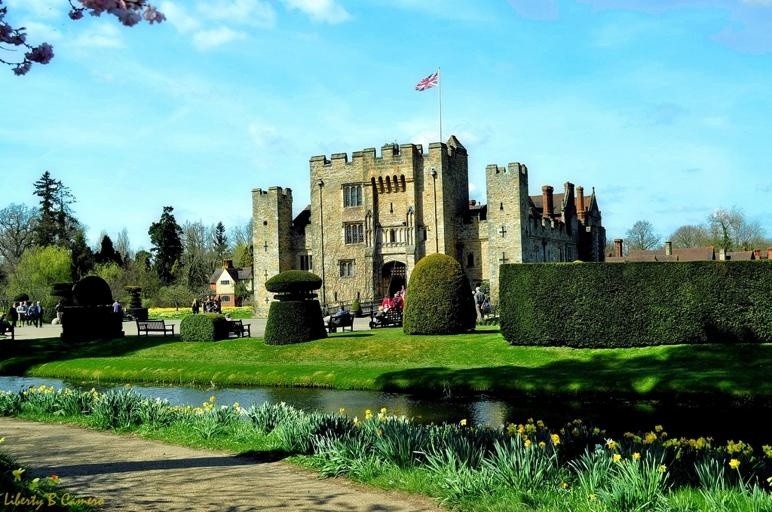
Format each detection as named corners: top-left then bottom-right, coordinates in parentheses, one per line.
top-left (188, 295), bottom-right (223, 315)
top-left (55, 299), bottom-right (64, 324)
top-left (323, 284), bottom-right (406, 332)
top-left (474, 286), bottom-right (484, 321)
top-left (113, 300), bottom-right (120, 312)
top-left (0, 299), bottom-right (45, 335)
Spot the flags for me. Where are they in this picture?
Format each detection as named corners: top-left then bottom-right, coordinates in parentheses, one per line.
top-left (415, 71), bottom-right (439, 92)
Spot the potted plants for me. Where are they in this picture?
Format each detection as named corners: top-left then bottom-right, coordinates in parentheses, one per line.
top-left (350, 298), bottom-right (363, 318)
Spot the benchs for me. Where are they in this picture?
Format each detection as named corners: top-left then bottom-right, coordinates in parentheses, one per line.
top-left (324, 314), bottom-right (355, 332)
top-left (135, 319), bottom-right (175, 337)
top-left (227, 319), bottom-right (251, 337)
top-left (371, 306), bottom-right (403, 328)
top-left (479, 304), bottom-right (497, 318)
